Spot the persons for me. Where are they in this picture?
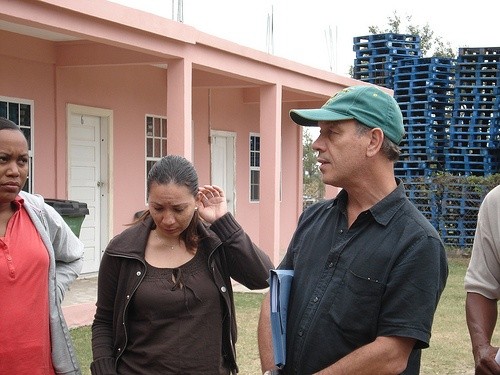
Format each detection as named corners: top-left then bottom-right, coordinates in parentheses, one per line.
top-left (257, 84), bottom-right (449, 375)
top-left (0, 116), bottom-right (85, 375)
top-left (91, 155), bottom-right (275, 375)
top-left (464, 186), bottom-right (500, 375)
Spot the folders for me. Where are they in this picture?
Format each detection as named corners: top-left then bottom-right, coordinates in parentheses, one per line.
top-left (268, 268), bottom-right (295, 370)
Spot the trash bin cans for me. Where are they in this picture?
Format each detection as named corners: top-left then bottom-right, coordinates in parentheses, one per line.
top-left (42, 197), bottom-right (90, 242)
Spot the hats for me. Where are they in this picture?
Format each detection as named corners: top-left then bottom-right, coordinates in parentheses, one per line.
top-left (289, 86), bottom-right (405, 145)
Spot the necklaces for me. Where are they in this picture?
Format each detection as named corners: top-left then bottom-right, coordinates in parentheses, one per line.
top-left (153, 230), bottom-right (179, 250)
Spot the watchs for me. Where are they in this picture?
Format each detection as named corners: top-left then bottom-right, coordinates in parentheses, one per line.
top-left (263, 368), bottom-right (278, 375)
